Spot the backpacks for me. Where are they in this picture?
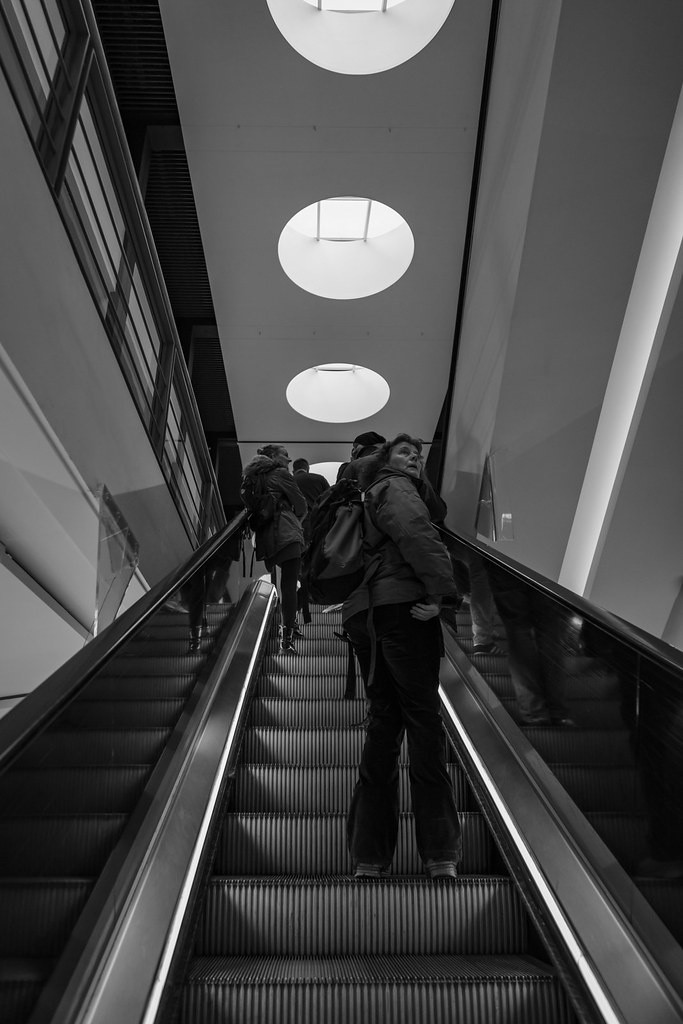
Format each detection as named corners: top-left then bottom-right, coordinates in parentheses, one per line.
top-left (239, 466), bottom-right (281, 534)
top-left (300, 476), bottom-right (367, 606)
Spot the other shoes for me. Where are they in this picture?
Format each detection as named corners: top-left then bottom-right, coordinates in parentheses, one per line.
top-left (424, 856), bottom-right (458, 880)
top-left (520, 711), bottom-right (552, 725)
top-left (554, 710), bottom-right (578, 726)
top-left (166, 600), bottom-right (189, 614)
top-left (350, 855), bottom-right (392, 877)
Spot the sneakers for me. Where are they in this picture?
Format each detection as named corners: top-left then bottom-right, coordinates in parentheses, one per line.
top-left (473, 640), bottom-right (511, 657)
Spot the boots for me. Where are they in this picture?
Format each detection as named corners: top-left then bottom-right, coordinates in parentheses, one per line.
top-left (202, 624), bottom-right (210, 636)
top-left (187, 626), bottom-right (202, 657)
top-left (294, 618), bottom-right (306, 638)
top-left (280, 626), bottom-right (299, 655)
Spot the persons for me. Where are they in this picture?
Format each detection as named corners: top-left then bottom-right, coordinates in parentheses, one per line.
top-left (187, 517), bottom-right (244, 654)
top-left (245, 445), bottom-right (331, 653)
top-left (337, 431), bottom-right (461, 879)
top-left (468, 562), bottom-right (569, 729)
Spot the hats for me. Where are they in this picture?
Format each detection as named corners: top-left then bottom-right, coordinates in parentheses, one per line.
top-left (353, 431), bottom-right (386, 447)
